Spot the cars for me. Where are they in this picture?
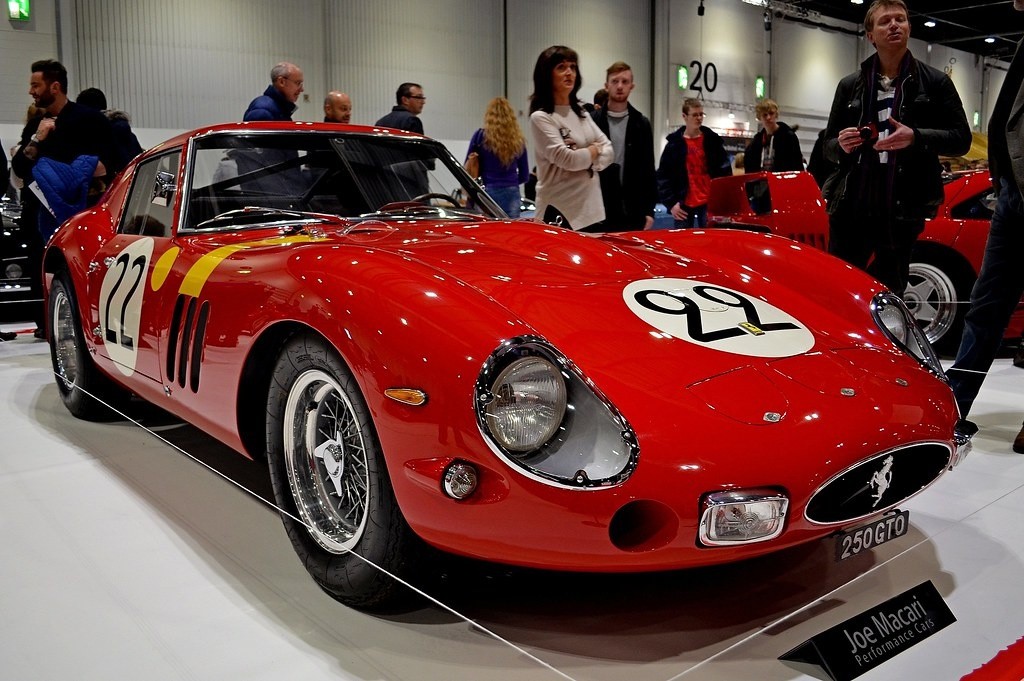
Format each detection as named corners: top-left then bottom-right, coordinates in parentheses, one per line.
top-left (0, 201), bottom-right (30, 301)
top-left (43, 122), bottom-right (980, 606)
top-left (705, 168), bottom-right (1024, 355)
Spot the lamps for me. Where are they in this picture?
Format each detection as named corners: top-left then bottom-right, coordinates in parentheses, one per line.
top-left (697, 0), bottom-right (705, 16)
top-left (764, 12), bottom-right (773, 31)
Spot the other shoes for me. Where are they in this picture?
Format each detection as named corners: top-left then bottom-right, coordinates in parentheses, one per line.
top-left (0, 330), bottom-right (16, 341)
top-left (1013, 422), bottom-right (1024, 454)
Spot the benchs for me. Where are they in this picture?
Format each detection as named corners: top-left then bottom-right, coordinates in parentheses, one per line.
top-left (183, 194), bottom-right (354, 229)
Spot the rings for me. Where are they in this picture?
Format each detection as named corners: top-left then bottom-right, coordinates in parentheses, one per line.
top-left (891, 146), bottom-right (893, 150)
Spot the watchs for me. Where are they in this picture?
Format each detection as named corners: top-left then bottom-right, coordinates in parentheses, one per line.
top-left (31, 134), bottom-right (39, 143)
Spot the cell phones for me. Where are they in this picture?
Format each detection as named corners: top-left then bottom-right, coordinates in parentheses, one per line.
top-left (559, 128), bottom-right (571, 147)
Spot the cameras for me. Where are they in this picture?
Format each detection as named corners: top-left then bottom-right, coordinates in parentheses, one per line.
top-left (858, 122), bottom-right (879, 142)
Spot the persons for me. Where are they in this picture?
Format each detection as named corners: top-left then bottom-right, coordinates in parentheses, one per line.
top-left (463, 97), bottom-right (529, 219)
top-left (950, 0), bottom-right (1024, 456)
top-left (11, 60), bottom-right (146, 339)
top-left (375, 83), bottom-right (436, 204)
top-left (656, 97), bottom-right (734, 229)
top-left (212, 62), bottom-right (306, 209)
top-left (732, 98), bottom-right (805, 233)
top-left (525, 61), bottom-right (658, 232)
top-left (524, 45), bottom-right (614, 233)
top-left (305, 90), bottom-right (355, 216)
top-left (804, 0), bottom-right (973, 298)
top-left (0, 137), bottom-right (18, 342)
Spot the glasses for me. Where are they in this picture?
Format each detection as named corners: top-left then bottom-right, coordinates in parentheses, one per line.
top-left (406, 95), bottom-right (426, 101)
top-left (684, 112), bottom-right (708, 118)
